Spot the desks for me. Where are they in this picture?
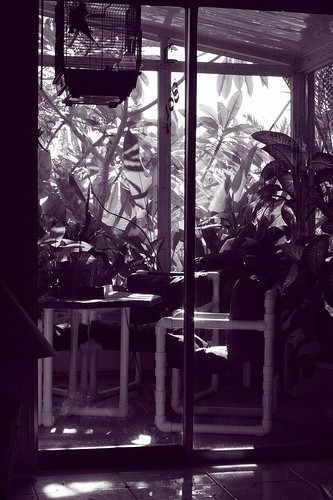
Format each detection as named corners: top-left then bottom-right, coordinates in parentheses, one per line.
top-left (35, 290), bottom-right (164, 427)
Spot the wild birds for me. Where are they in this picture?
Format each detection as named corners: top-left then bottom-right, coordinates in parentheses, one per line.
top-left (67, 0), bottom-right (101, 49)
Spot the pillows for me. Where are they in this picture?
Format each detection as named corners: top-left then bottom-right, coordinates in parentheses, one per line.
top-left (222, 269), bottom-right (280, 362)
top-left (125, 273), bottom-right (212, 348)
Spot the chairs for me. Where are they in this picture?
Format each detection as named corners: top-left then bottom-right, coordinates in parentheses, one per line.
top-left (151, 260), bottom-right (301, 434)
top-left (83, 266), bottom-right (224, 422)
